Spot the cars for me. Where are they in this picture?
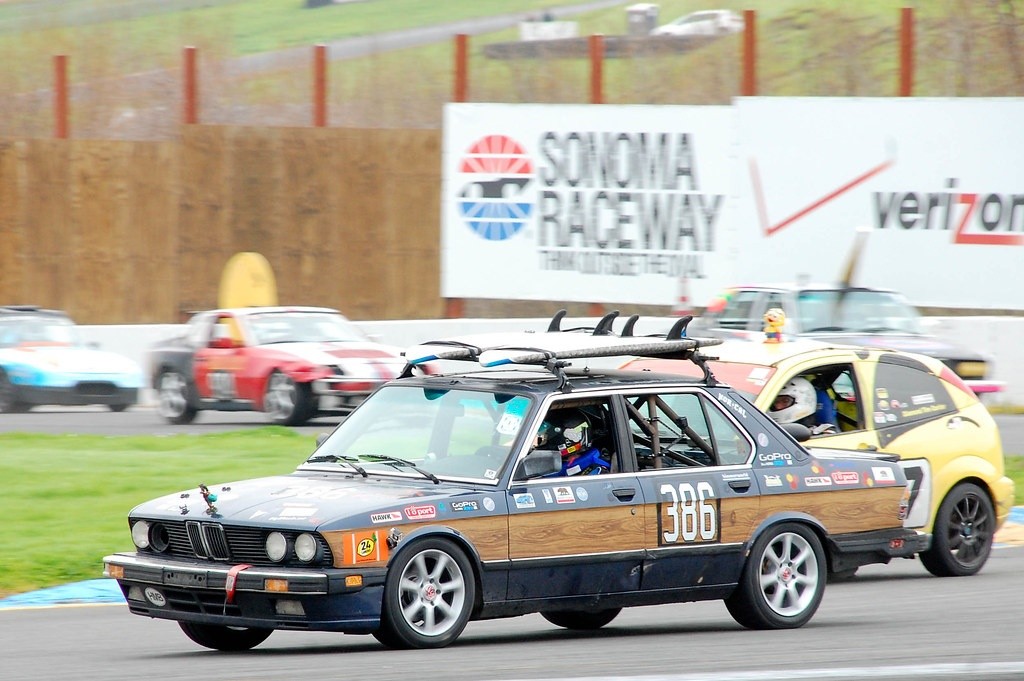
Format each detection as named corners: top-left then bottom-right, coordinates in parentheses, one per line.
top-left (689, 282), bottom-right (1003, 407)
top-left (144, 303), bottom-right (438, 427)
top-left (0, 304), bottom-right (147, 413)
top-left (104, 310), bottom-right (932, 653)
top-left (474, 311), bottom-right (1017, 582)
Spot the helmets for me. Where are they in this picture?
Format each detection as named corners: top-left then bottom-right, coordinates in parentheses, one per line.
top-left (769, 375), bottom-right (817, 424)
top-left (535, 407), bottom-right (594, 457)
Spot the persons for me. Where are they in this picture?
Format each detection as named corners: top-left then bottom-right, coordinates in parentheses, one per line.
top-left (528, 407), bottom-right (609, 479)
top-left (767, 377), bottom-right (835, 435)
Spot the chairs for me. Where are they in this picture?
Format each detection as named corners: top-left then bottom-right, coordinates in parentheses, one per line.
top-left (587, 404), bottom-right (615, 463)
top-left (816, 389), bottom-right (836, 424)
